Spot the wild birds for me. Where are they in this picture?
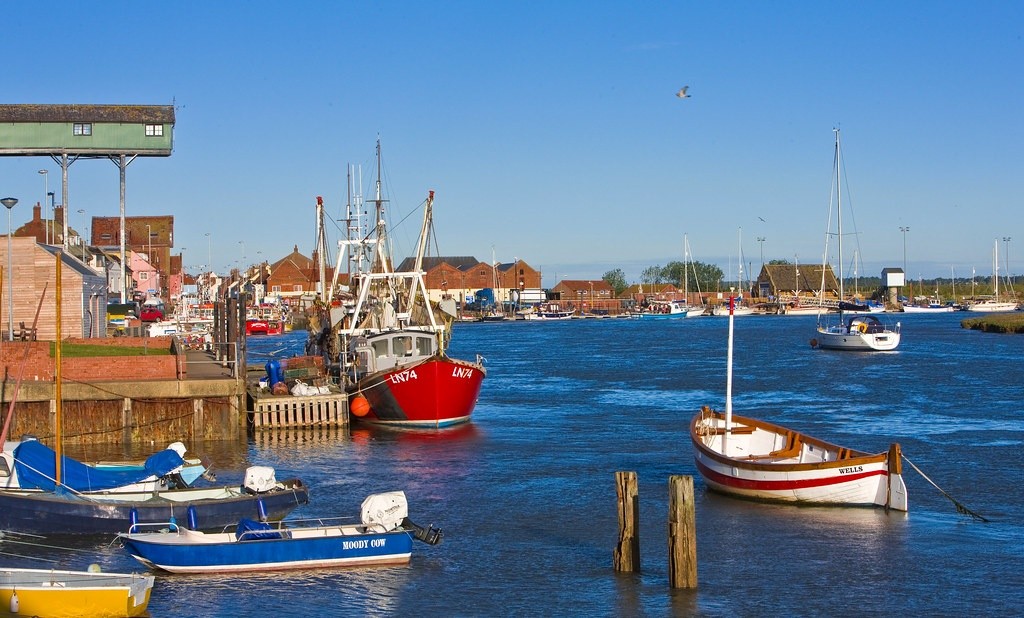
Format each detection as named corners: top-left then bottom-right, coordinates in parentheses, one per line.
top-left (672, 86), bottom-right (692, 98)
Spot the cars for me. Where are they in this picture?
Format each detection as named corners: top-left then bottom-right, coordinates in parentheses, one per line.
top-left (140, 308), bottom-right (163, 323)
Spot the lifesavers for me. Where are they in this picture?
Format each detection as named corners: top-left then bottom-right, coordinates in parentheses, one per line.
top-left (857, 323), bottom-right (867, 334)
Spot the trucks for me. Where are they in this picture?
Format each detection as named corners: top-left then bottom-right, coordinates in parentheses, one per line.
top-left (105, 305), bottom-right (127, 330)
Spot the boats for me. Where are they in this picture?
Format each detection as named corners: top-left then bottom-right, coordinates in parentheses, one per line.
top-left (310, 131), bottom-right (486, 428)
top-left (144, 301), bottom-right (293, 343)
top-left (689, 286), bottom-right (908, 512)
top-left (1, 250), bottom-right (412, 618)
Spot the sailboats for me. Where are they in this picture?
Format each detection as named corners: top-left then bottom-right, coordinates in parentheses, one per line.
top-left (630, 225), bottom-right (828, 319)
top-left (454, 248), bottom-right (577, 322)
top-left (901, 265), bottom-right (967, 314)
top-left (811, 122), bottom-right (901, 351)
top-left (968, 237), bottom-right (1019, 312)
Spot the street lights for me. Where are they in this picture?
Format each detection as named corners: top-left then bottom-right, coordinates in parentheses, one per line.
top-left (205, 231), bottom-right (211, 272)
top-left (37, 169), bottom-right (49, 245)
top-left (77, 209), bottom-right (85, 262)
top-left (0, 197), bottom-right (19, 341)
top-left (1003, 237), bottom-right (1011, 285)
top-left (145, 224), bottom-right (151, 265)
top-left (899, 226), bottom-right (910, 285)
top-left (560, 274), bottom-right (568, 292)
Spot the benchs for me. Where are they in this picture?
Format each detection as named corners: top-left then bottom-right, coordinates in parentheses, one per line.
top-left (731, 455), bottom-right (793, 462)
